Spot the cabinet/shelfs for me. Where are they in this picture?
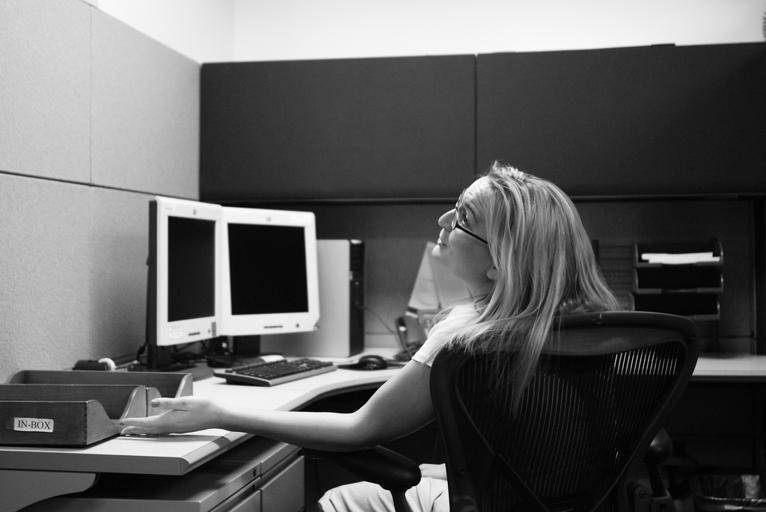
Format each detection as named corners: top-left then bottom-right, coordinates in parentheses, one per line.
top-left (198, 40), bottom-right (766, 204)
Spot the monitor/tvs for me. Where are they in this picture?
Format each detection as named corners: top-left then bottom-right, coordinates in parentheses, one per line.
top-left (128, 195), bottom-right (223, 381)
top-left (208, 207), bottom-right (319, 367)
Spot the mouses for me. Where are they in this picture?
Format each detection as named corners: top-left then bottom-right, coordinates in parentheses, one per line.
top-left (359, 355), bottom-right (387, 368)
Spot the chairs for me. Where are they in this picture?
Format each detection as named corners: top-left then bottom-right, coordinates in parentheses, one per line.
top-left (299, 308), bottom-right (699, 512)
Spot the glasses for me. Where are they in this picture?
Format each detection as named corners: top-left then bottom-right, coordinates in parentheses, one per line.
top-left (451, 201), bottom-right (489, 246)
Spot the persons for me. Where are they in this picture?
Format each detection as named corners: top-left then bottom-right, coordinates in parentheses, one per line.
top-left (119, 160), bottom-right (624, 512)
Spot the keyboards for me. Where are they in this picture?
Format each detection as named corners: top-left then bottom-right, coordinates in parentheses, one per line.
top-left (215, 358), bottom-right (336, 386)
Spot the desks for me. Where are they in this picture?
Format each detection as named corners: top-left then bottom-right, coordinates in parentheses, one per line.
top-left (0, 347), bottom-right (765, 512)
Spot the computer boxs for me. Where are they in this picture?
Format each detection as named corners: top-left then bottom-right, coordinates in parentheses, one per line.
top-left (228, 237), bottom-right (363, 358)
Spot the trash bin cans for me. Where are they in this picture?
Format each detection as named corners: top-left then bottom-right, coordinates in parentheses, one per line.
top-left (694, 474), bottom-right (766, 512)
